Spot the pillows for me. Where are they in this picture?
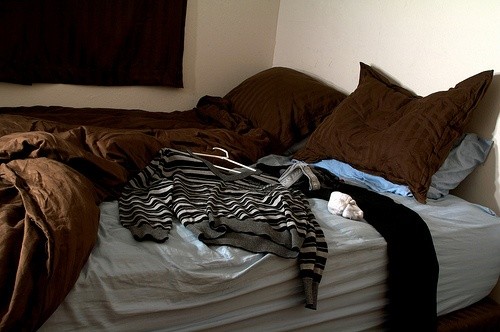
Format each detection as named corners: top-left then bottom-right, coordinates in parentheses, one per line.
top-left (224, 65), bottom-right (349, 147)
top-left (317, 130), bottom-right (494, 199)
top-left (292, 62), bottom-right (497, 204)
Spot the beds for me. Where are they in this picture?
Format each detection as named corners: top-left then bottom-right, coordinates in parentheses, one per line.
top-left (0, 102), bottom-right (500, 332)
